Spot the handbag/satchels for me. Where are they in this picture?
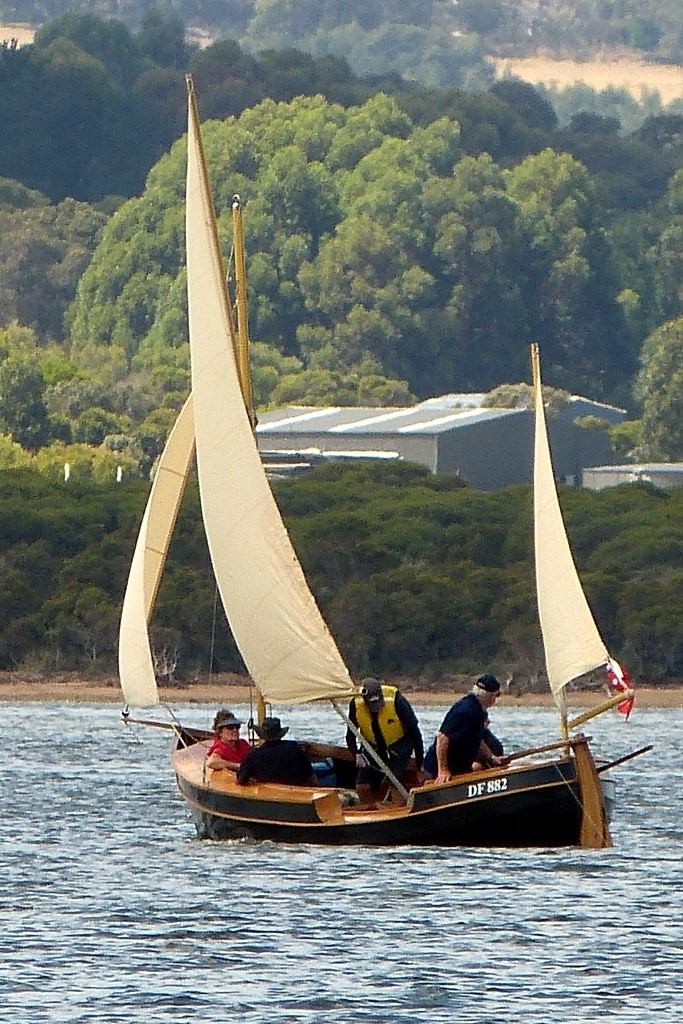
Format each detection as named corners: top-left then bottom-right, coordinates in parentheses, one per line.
top-left (309, 755), bottom-right (336, 787)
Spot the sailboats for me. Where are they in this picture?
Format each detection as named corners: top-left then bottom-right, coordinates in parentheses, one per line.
top-left (104, 73), bottom-right (657, 845)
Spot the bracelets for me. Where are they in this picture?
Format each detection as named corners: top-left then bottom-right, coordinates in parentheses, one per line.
top-left (489, 755), bottom-right (495, 764)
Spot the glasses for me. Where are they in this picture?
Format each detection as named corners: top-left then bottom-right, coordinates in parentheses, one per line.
top-left (495, 692), bottom-right (500, 697)
top-left (226, 724), bottom-right (240, 730)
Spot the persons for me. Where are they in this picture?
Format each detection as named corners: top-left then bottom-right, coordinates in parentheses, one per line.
top-left (422, 674), bottom-right (510, 783)
top-left (206, 710), bottom-right (252, 771)
top-left (346, 678), bottom-right (428, 805)
top-left (236, 718), bottom-right (318, 786)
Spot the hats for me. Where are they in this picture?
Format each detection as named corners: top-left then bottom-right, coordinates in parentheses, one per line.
top-left (217, 711), bottom-right (241, 728)
top-left (474, 674), bottom-right (500, 692)
top-left (361, 678), bottom-right (383, 712)
top-left (253, 717), bottom-right (289, 742)
